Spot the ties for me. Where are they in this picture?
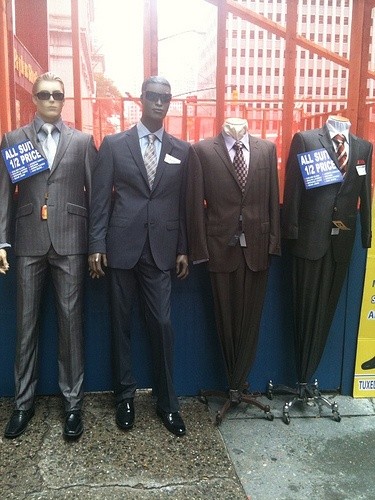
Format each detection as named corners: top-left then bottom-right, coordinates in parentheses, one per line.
top-left (333, 134), bottom-right (348, 192)
top-left (40, 123), bottom-right (57, 170)
top-left (143, 134), bottom-right (158, 190)
top-left (232, 142), bottom-right (248, 201)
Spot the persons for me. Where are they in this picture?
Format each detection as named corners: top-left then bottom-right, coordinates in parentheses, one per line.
top-left (87, 76), bottom-right (189, 437)
top-left (281, 116), bottom-right (373, 397)
top-left (0, 73), bottom-right (99, 439)
top-left (193, 118), bottom-right (281, 403)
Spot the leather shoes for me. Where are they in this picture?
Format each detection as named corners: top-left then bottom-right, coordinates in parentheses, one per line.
top-left (115, 400), bottom-right (134, 432)
top-left (158, 407), bottom-right (186, 436)
top-left (4, 407), bottom-right (35, 439)
top-left (64, 410), bottom-right (83, 438)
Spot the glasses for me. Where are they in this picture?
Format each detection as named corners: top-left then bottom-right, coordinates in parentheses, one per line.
top-left (34, 92), bottom-right (64, 101)
top-left (146, 91), bottom-right (172, 104)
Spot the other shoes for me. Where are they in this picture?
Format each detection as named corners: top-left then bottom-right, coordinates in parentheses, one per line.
top-left (361, 355), bottom-right (375, 370)
